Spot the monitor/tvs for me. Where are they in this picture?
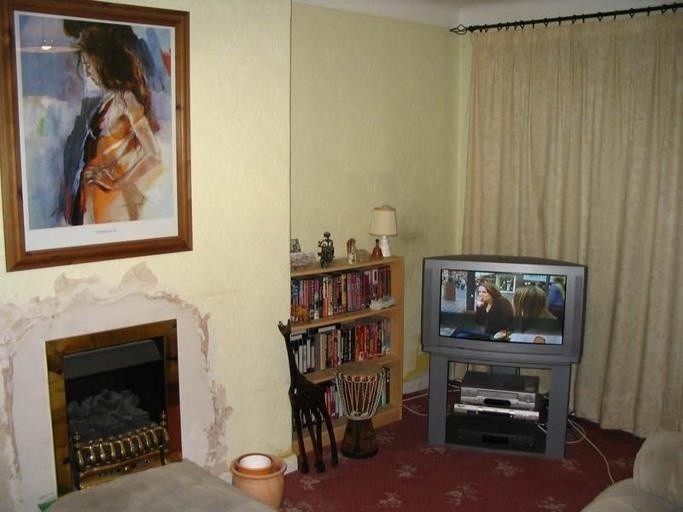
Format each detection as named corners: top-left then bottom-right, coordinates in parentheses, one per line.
top-left (421, 255), bottom-right (589, 365)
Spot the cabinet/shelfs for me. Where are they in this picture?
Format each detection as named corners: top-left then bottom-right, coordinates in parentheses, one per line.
top-left (289, 250), bottom-right (405, 459)
top-left (426, 354), bottom-right (573, 463)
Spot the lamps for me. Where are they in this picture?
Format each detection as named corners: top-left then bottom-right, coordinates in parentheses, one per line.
top-left (367, 203), bottom-right (400, 258)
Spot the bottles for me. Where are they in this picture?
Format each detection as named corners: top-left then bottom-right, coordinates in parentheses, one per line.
top-left (370, 239), bottom-right (383, 260)
top-left (346, 238), bottom-right (357, 264)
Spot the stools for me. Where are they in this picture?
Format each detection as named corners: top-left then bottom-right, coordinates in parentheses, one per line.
top-left (334, 361), bottom-right (387, 459)
top-left (43, 457), bottom-right (280, 510)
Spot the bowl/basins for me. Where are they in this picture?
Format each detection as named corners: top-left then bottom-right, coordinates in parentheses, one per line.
top-left (235, 452), bottom-right (277, 476)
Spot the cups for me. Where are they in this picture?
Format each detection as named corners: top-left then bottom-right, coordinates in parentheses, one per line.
top-left (229, 460), bottom-right (288, 512)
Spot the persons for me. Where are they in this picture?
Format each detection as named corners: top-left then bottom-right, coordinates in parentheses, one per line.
top-left (71, 25), bottom-right (159, 229)
top-left (474, 278), bottom-right (566, 342)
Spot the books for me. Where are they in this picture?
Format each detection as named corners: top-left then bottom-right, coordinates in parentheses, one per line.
top-left (290, 265), bottom-right (392, 431)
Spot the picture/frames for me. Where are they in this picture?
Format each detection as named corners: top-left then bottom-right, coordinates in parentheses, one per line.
top-left (0, 1), bottom-right (193, 275)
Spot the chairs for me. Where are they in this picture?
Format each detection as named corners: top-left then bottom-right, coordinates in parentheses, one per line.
top-left (584, 428), bottom-right (682, 511)
